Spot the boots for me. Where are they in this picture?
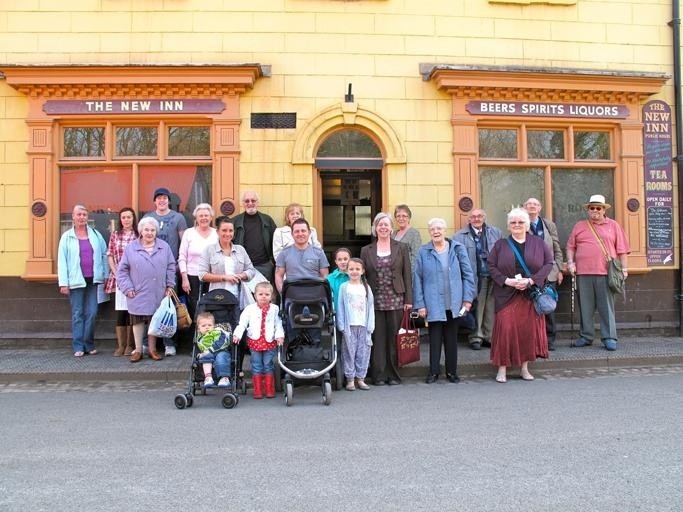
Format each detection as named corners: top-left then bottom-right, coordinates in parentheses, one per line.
top-left (263, 374), bottom-right (277, 398)
top-left (251, 373), bottom-right (264, 399)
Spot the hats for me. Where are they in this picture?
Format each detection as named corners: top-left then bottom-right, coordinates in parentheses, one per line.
top-left (583, 194), bottom-right (612, 209)
top-left (153, 188), bottom-right (171, 201)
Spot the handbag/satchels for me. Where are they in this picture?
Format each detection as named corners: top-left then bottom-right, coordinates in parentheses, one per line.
top-left (396, 309), bottom-right (420, 369)
top-left (167, 287), bottom-right (192, 331)
top-left (506, 237), bottom-right (559, 315)
top-left (587, 219), bottom-right (626, 294)
top-left (92, 228), bottom-right (111, 304)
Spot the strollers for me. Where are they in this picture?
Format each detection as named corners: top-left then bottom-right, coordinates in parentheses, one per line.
top-left (277, 279), bottom-right (338, 406)
top-left (175, 288), bottom-right (240, 408)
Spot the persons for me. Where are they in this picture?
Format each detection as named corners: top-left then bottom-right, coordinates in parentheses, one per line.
top-left (177, 203), bottom-right (219, 347)
top-left (198, 216), bottom-right (255, 303)
top-left (336, 258), bottom-right (375, 392)
top-left (327, 248), bottom-right (351, 314)
top-left (105, 207), bottom-right (140, 357)
top-left (232, 281), bottom-right (285, 399)
top-left (565, 194), bottom-right (631, 352)
top-left (390, 205), bottom-right (422, 285)
top-left (485, 207), bottom-right (553, 383)
top-left (230, 191), bottom-right (277, 285)
top-left (275, 218), bottom-right (329, 298)
top-left (412, 218), bottom-right (475, 384)
top-left (116, 216), bottom-right (176, 363)
top-left (522, 197), bottom-right (563, 352)
top-left (137, 188), bottom-right (188, 358)
top-left (272, 202), bottom-right (324, 265)
top-left (57, 204), bottom-right (110, 357)
top-left (360, 211), bottom-right (412, 386)
top-left (195, 312), bottom-right (233, 388)
top-left (451, 209), bottom-right (504, 351)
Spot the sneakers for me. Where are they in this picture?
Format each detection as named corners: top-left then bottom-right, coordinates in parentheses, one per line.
top-left (143, 345), bottom-right (150, 355)
top-left (149, 351), bottom-right (162, 360)
top-left (470, 341), bottom-right (482, 350)
top-left (375, 378), bottom-right (385, 386)
top-left (496, 371), bottom-right (507, 383)
top-left (130, 352), bottom-right (143, 362)
top-left (124, 344), bottom-right (135, 356)
top-left (520, 370), bottom-right (534, 381)
top-left (605, 339), bottom-right (617, 351)
top-left (574, 337), bottom-right (592, 347)
top-left (112, 343), bottom-right (123, 357)
top-left (218, 377), bottom-right (230, 388)
top-left (426, 373), bottom-right (439, 384)
top-left (346, 380), bottom-right (355, 391)
top-left (165, 345), bottom-right (176, 357)
top-left (358, 382), bottom-right (371, 390)
top-left (89, 349), bottom-right (96, 355)
top-left (548, 341), bottom-right (555, 351)
top-left (389, 379), bottom-right (399, 385)
top-left (74, 350), bottom-right (84, 357)
top-left (204, 376), bottom-right (214, 387)
top-left (446, 371), bottom-right (460, 383)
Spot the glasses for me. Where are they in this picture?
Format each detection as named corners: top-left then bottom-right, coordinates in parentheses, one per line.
top-left (590, 207), bottom-right (602, 211)
top-left (244, 199), bottom-right (257, 204)
top-left (510, 221), bottom-right (525, 225)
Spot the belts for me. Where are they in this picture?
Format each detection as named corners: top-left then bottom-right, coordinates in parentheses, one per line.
top-left (479, 275), bottom-right (492, 280)
top-left (253, 259), bottom-right (270, 266)
